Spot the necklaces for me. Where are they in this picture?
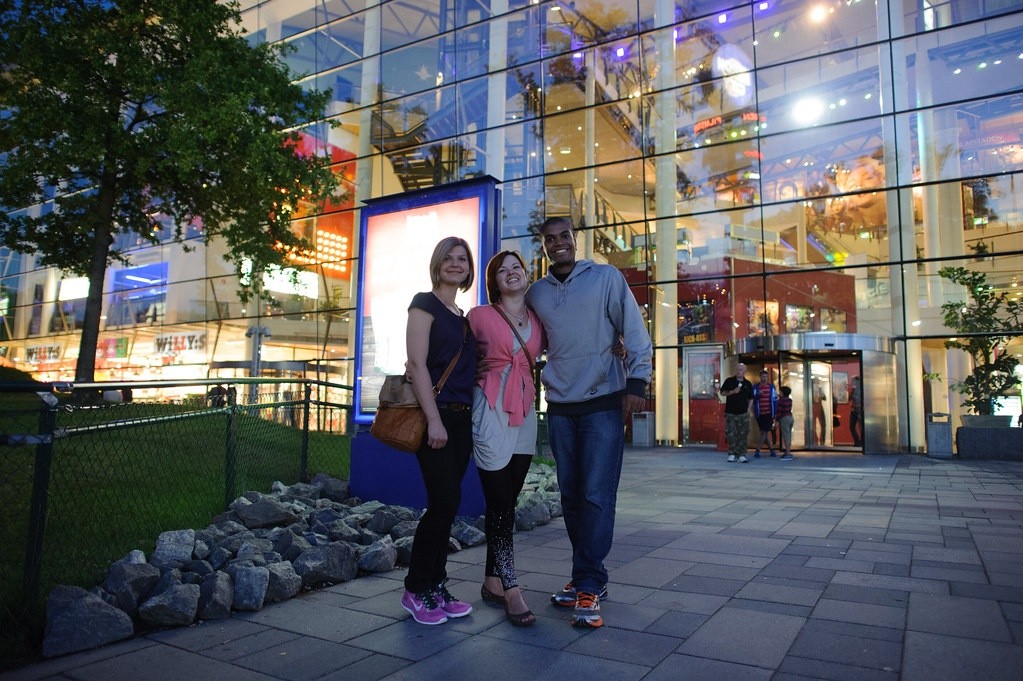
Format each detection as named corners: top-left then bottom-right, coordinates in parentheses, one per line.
top-left (504, 305), bottom-right (526, 326)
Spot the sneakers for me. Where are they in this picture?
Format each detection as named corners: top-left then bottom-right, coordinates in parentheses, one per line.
top-left (570, 591), bottom-right (602, 627)
top-left (551, 580), bottom-right (609, 608)
top-left (401, 589), bottom-right (448, 625)
top-left (727, 454), bottom-right (735, 462)
top-left (739, 456), bottom-right (748, 463)
top-left (430, 585), bottom-right (472, 618)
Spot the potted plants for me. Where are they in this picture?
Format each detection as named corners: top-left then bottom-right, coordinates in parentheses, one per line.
top-left (940, 267), bottom-right (1023, 427)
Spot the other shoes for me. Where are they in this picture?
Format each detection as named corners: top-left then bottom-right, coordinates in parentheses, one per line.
top-left (770, 450), bottom-right (776, 457)
top-left (779, 455), bottom-right (793, 461)
top-left (755, 449), bottom-right (760, 457)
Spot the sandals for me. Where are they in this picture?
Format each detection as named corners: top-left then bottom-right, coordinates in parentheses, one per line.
top-left (504, 598), bottom-right (536, 626)
top-left (482, 584), bottom-right (507, 606)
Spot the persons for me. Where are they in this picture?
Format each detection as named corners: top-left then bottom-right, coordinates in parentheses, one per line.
top-left (720, 363), bottom-right (755, 463)
top-left (207, 385), bottom-right (227, 408)
top-left (848, 375), bottom-right (864, 447)
top-left (405, 250), bottom-right (626, 625)
top-left (810, 375), bottom-right (826, 445)
top-left (774, 385), bottom-right (795, 460)
top-left (751, 371), bottom-right (778, 457)
top-left (401, 235), bottom-right (485, 623)
top-left (521, 220), bottom-right (654, 627)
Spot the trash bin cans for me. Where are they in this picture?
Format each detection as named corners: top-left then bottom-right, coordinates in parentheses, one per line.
top-left (926, 412), bottom-right (953, 459)
top-left (631, 411), bottom-right (655, 447)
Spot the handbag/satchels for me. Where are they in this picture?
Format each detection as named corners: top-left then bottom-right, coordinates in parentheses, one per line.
top-left (370, 375), bottom-right (438, 451)
